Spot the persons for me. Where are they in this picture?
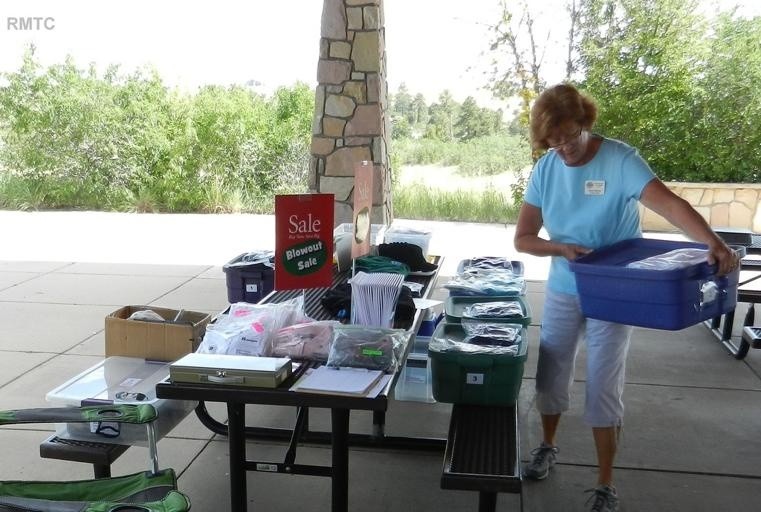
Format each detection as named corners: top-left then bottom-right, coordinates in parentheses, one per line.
top-left (511, 82), bottom-right (741, 512)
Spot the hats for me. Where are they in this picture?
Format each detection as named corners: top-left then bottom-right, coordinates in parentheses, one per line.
top-left (378, 243), bottom-right (438, 272)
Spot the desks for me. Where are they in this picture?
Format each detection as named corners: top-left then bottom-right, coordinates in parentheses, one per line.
top-left (159, 252), bottom-right (445, 512)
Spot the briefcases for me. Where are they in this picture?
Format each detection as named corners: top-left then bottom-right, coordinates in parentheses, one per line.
top-left (169, 352), bottom-right (291, 389)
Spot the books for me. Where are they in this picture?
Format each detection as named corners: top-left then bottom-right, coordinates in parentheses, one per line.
top-left (347, 270), bottom-right (406, 329)
top-left (295, 365), bottom-right (387, 398)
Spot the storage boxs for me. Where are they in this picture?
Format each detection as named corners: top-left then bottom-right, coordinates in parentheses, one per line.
top-left (46, 221), bottom-right (533, 447)
top-left (569, 236), bottom-right (746, 333)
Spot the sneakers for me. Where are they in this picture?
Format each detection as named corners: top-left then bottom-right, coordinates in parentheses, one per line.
top-left (583, 485), bottom-right (619, 512)
top-left (523, 443), bottom-right (559, 480)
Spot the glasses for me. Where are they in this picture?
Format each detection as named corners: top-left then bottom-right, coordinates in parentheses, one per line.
top-left (548, 129), bottom-right (582, 152)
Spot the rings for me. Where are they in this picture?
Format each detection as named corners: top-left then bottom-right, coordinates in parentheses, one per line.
top-left (731, 264), bottom-right (737, 268)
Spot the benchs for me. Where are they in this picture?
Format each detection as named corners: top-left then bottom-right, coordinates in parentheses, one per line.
top-left (439, 403), bottom-right (523, 511)
